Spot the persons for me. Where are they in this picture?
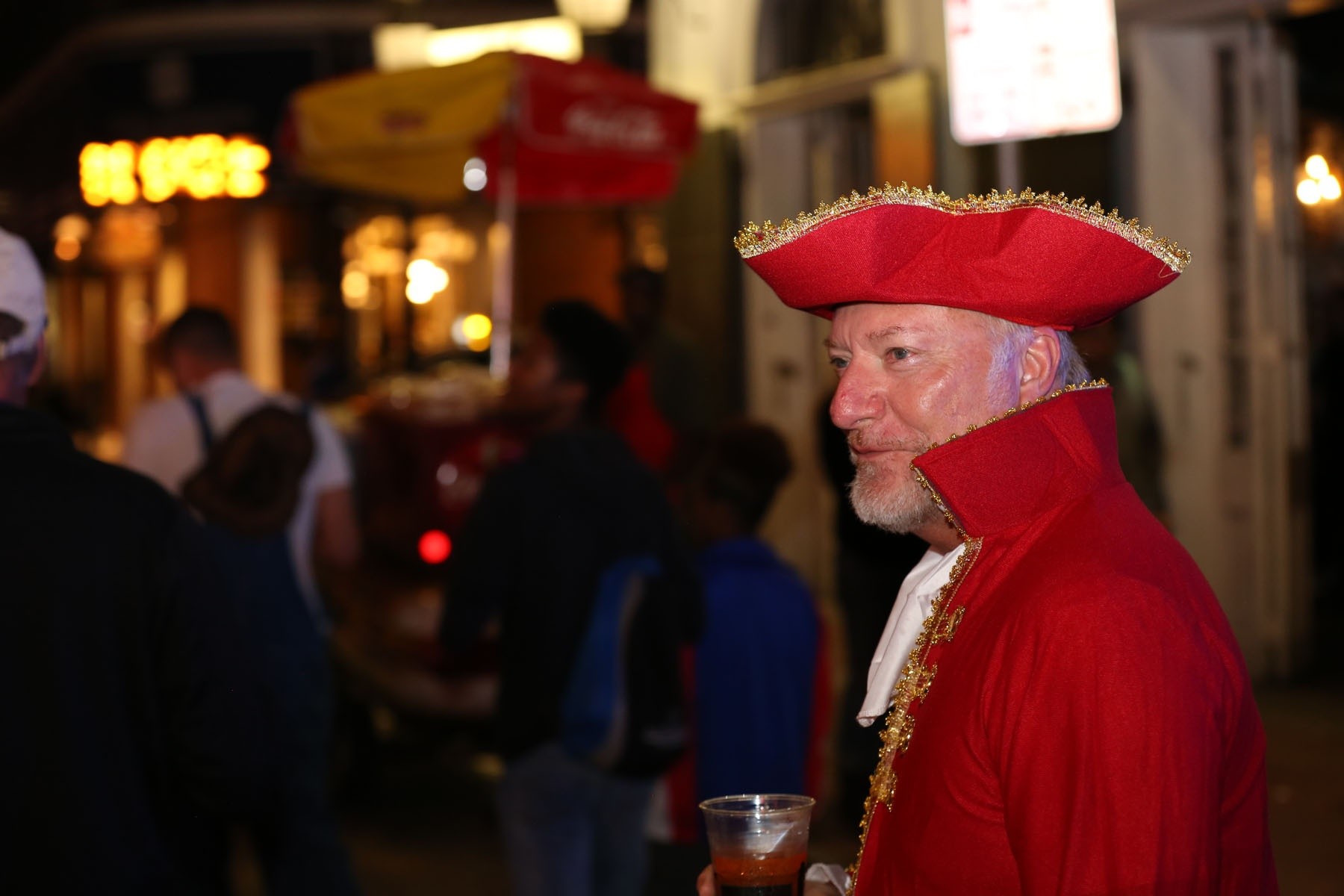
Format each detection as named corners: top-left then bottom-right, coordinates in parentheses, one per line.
top-left (694, 182), bottom-right (1279, 896)
top-left (0, 234), bottom-right (1156, 896)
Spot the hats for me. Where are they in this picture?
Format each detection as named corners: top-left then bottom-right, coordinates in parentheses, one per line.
top-left (0, 231), bottom-right (47, 361)
top-left (732, 182), bottom-right (1192, 330)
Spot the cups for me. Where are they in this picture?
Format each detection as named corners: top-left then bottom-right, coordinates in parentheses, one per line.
top-left (698, 792), bottom-right (817, 895)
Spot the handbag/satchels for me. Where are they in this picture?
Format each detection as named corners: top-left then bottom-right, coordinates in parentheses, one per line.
top-left (177, 391), bottom-right (320, 544)
